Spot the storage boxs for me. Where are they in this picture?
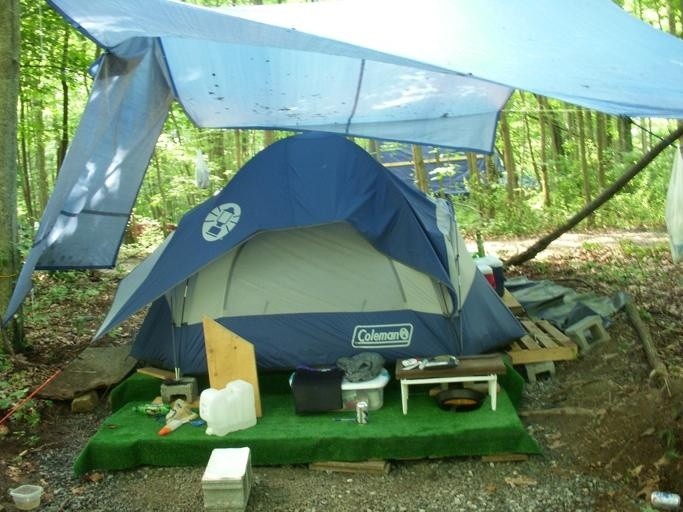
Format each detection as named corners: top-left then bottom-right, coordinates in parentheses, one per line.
top-left (286, 363), bottom-right (393, 414)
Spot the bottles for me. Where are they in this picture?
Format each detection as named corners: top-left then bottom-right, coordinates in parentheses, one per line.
top-left (475, 231), bottom-right (485, 256)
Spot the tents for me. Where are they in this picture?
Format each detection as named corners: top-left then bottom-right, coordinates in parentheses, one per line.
top-left (86, 130), bottom-right (527, 376)
top-left (365, 137), bottom-right (536, 198)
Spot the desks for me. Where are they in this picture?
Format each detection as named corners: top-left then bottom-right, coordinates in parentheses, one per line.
top-left (394, 355), bottom-right (509, 415)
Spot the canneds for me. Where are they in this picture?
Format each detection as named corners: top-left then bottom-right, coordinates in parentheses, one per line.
top-left (356, 401), bottom-right (368, 425)
top-left (651, 491), bottom-right (680, 508)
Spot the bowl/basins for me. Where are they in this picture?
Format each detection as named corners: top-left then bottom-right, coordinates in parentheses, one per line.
top-left (10, 484), bottom-right (42, 510)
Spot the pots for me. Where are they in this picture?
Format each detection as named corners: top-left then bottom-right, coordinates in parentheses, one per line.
top-left (435, 385), bottom-right (487, 414)
